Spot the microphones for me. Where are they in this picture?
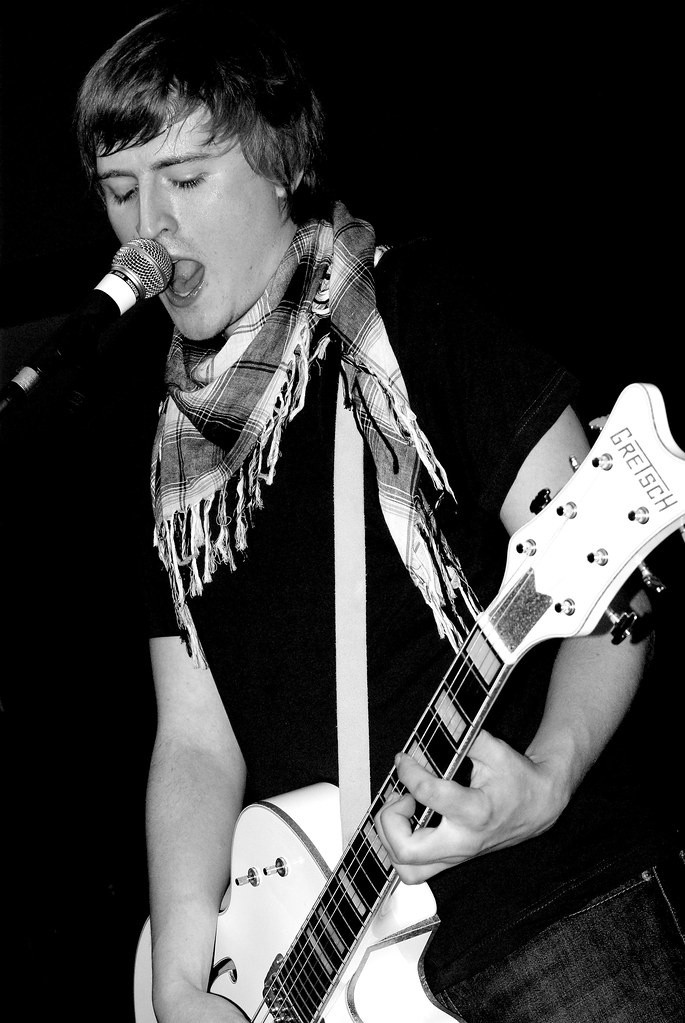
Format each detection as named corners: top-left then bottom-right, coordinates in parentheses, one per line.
top-left (3, 239), bottom-right (173, 400)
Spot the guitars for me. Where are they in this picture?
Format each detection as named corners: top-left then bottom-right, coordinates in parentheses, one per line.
top-left (131, 379), bottom-right (684, 1022)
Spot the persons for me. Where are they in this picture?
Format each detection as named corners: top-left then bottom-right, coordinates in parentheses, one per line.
top-left (81, 0), bottom-right (685, 1023)
